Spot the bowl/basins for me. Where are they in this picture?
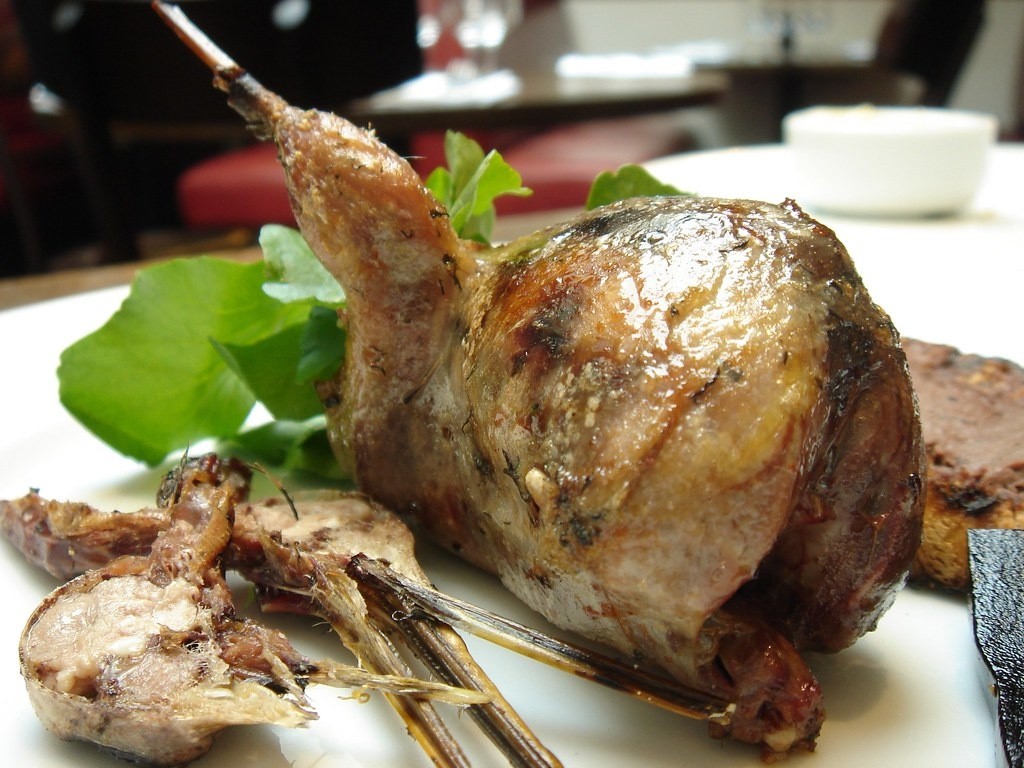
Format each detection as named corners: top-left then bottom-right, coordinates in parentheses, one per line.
top-left (783, 102), bottom-right (1000, 219)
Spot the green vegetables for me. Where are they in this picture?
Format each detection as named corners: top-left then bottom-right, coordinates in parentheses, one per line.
top-left (56, 130), bottom-right (688, 480)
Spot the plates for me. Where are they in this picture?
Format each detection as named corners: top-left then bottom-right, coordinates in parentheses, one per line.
top-left (0, 275), bottom-right (1024, 768)
top-left (648, 138), bottom-right (1022, 283)
